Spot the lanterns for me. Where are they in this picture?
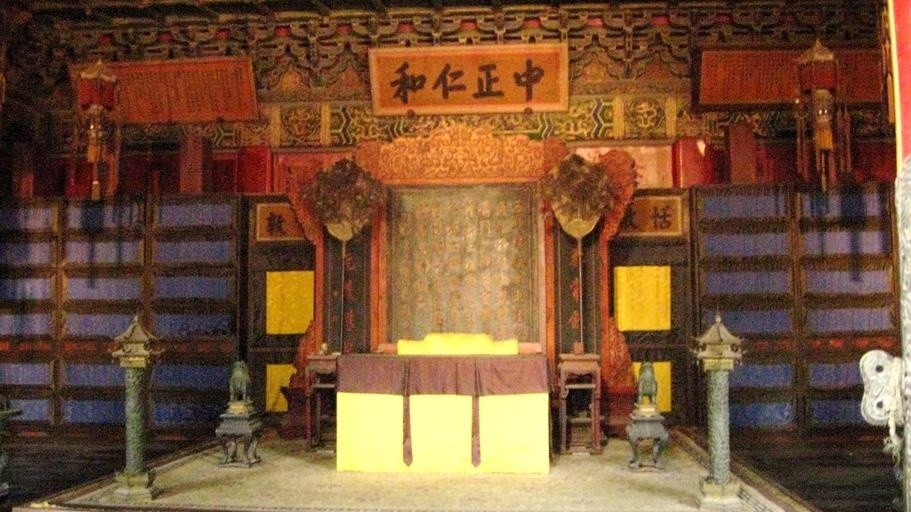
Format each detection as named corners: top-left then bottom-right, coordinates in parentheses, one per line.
top-left (71, 58), bottom-right (121, 202)
top-left (796, 40), bottom-right (846, 195)
